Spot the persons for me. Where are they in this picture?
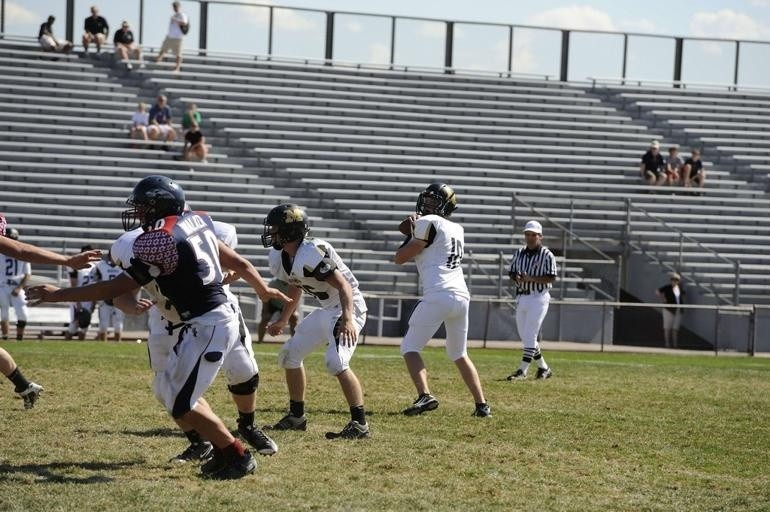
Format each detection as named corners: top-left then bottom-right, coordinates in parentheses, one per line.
top-left (655, 271), bottom-right (685, 348)
top-left (395, 182), bottom-right (493, 419)
top-left (38, 15), bottom-right (74, 53)
top-left (130, 92), bottom-right (208, 163)
top-left (113, 20), bottom-right (146, 69)
top-left (155, 1), bottom-right (188, 72)
top-left (506, 220), bottom-right (557, 381)
top-left (1, 173), bottom-right (298, 480)
top-left (82, 7), bottom-right (109, 55)
top-left (262, 203), bottom-right (371, 441)
top-left (638, 139), bottom-right (707, 188)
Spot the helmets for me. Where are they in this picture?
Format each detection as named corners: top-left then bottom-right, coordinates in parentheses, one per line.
top-left (6, 228), bottom-right (19, 239)
top-left (261, 203), bottom-right (310, 250)
top-left (122, 175), bottom-right (185, 232)
top-left (416, 183), bottom-right (458, 218)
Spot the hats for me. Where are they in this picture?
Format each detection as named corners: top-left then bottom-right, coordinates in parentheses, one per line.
top-left (522, 221), bottom-right (542, 235)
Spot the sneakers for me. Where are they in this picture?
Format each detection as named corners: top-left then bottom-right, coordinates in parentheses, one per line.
top-left (169, 414), bottom-right (306, 480)
top-left (507, 369), bottom-right (527, 381)
top-left (535, 368), bottom-right (552, 380)
top-left (15, 382), bottom-right (42, 410)
top-left (402, 394), bottom-right (439, 415)
top-left (326, 420), bottom-right (369, 440)
top-left (471, 405), bottom-right (490, 416)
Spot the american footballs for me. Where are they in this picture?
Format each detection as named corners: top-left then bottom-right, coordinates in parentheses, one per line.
top-left (399, 219), bottom-right (414, 237)
